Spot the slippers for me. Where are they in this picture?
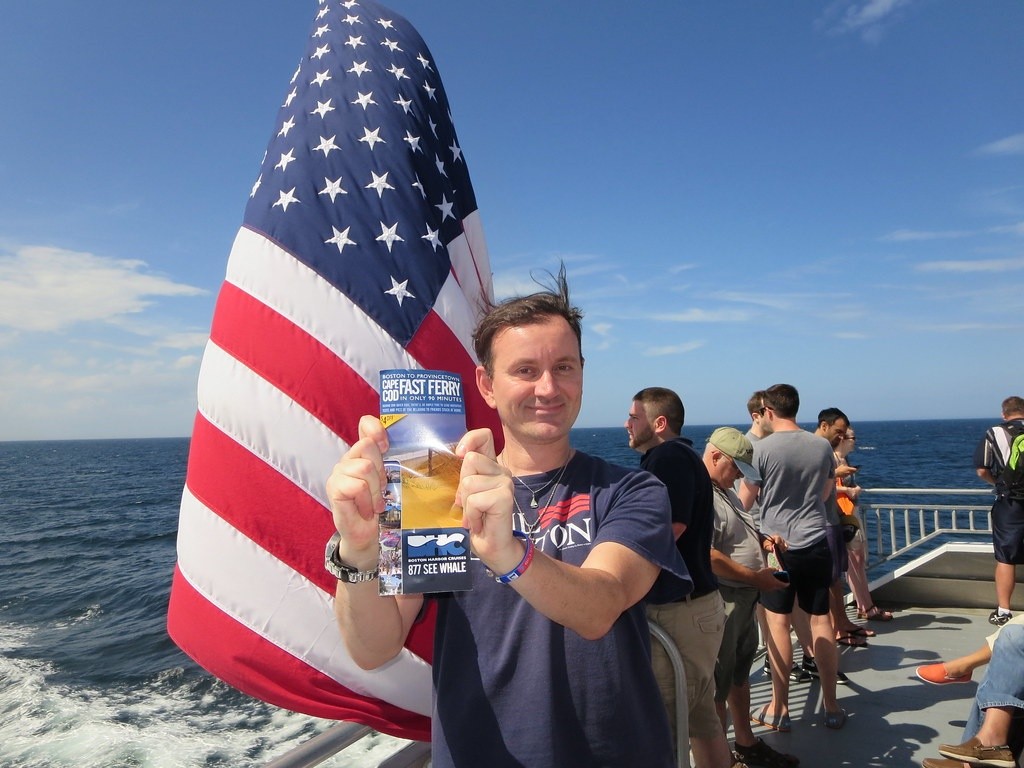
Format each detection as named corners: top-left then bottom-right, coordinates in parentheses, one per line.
top-left (846, 627), bottom-right (876, 637)
top-left (839, 634), bottom-right (867, 647)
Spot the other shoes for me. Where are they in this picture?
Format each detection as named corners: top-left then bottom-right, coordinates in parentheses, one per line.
top-left (916, 662), bottom-right (972, 684)
top-left (750, 705), bottom-right (790, 731)
top-left (922, 757), bottom-right (966, 768)
top-left (825, 706), bottom-right (845, 728)
top-left (939, 737), bottom-right (1017, 768)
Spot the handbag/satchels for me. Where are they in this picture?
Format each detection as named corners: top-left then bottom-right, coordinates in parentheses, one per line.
top-left (832, 523), bottom-right (857, 571)
top-left (832, 478), bottom-right (854, 516)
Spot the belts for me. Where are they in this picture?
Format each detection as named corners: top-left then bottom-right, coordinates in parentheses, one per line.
top-left (648, 586), bottom-right (720, 605)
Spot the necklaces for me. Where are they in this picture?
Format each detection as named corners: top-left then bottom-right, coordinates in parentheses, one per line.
top-left (501, 449), bottom-right (572, 548)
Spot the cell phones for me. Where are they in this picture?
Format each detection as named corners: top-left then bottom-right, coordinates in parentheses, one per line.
top-left (854, 464), bottom-right (861, 468)
top-left (772, 570), bottom-right (790, 583)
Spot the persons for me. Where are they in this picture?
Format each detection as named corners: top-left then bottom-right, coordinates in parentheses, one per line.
top-left (326, 292), bottom-right (693, 768)
top-left (701, 426), bottom-right (798, 768)
top-left (917, 613), bottom-right (1024, 768)
top-left (736, 383), bottom-right (893, 732)
top-left (625, 387), bottom-right (746, 768)
top-left (974, 396), bottom-right (1024, 625)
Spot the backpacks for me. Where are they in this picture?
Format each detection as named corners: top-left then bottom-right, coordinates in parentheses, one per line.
top-left (990, 421), bottom-right (1024, 499)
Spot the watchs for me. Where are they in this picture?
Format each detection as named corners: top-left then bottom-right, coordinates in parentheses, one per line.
top-left (324, 531), bottom-right (379, 582)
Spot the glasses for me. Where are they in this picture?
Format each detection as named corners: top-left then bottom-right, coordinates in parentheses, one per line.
top-left (758, 405), bottom-right (773, 416)
top-left (719, 452), bottom-right (743, 477)
top-left (844, 436), bottom-right (856, 441)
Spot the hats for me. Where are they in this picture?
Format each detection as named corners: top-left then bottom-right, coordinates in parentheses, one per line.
top-left (709, 426), bottom-right (762, 481)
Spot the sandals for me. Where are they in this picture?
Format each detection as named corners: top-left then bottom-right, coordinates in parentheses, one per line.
top-left (857, 605), bottom-right (893, 621)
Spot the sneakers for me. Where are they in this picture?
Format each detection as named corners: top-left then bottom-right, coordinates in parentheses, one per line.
top-left (800, 653), bottom-right (849, 684)
top-left (762, 655), bottom-right (809, 682)
top-left (733, 735), bottom-right (799, 768)
top-left (989, 608), bottom-right (1012, 625)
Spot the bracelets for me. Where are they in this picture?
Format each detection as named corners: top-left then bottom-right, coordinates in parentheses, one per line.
top-left (483, 529), bottom-right (534, 584)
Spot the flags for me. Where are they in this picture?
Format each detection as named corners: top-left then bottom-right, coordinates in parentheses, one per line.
top-left (167, 0), bottom-right (494, 743)
